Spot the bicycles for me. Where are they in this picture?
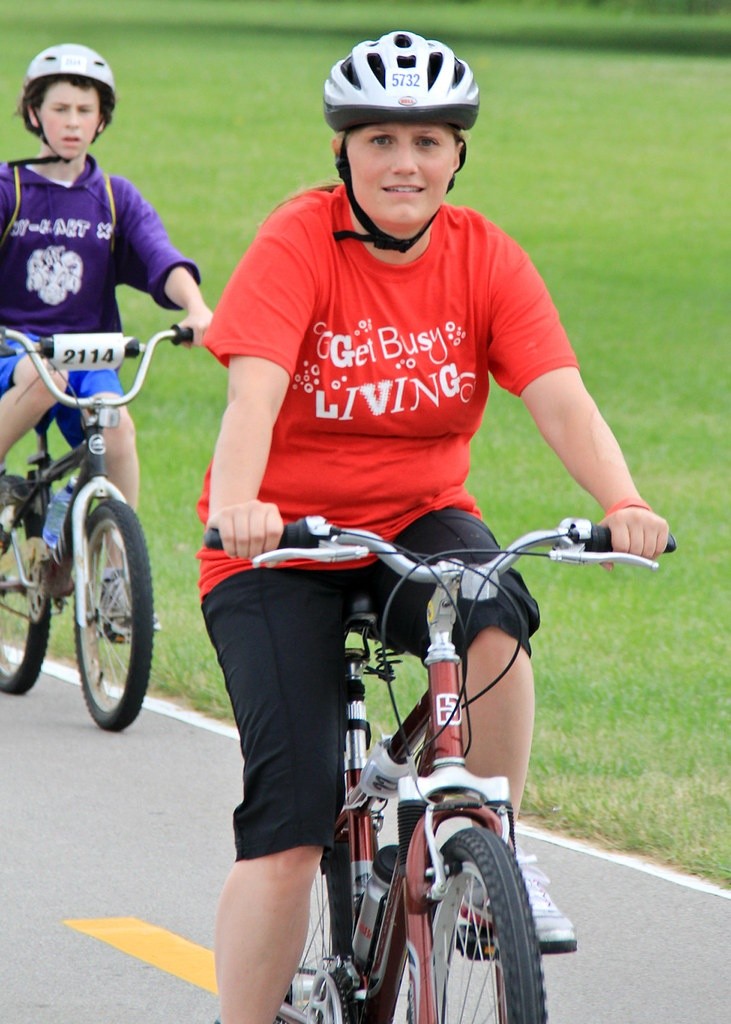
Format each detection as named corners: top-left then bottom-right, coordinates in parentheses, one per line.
top-left (203, 501), bottom-right (678, 1024)
top-left (0, 319), bottom-right (205, 733)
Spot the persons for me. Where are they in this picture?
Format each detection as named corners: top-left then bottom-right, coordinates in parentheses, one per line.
top-left (1, 42), bottom-right (216, 639)
top-left (195, 30), bottom-right (672, 1024)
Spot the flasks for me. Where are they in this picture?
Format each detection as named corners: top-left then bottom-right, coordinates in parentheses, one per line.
top-left (350, 845), bottom-right (401, 964)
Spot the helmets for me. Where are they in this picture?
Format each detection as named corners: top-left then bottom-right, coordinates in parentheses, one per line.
top-left (322, 30), bottom-right (481, 131)
top-left (25, 43), bottom-right (114, 96)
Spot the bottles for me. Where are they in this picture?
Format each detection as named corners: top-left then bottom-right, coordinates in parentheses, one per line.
top-left (38, 478), bottom-right (76, 549)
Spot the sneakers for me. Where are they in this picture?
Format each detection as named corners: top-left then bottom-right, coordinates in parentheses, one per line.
top-left (461, 852), bottom-right (578, 952)
top-left (99, 582), bottom-right (161, 633)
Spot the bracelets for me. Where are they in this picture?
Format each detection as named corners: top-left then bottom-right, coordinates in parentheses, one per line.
top-left (603, 497), bottom-right (652, 517)
